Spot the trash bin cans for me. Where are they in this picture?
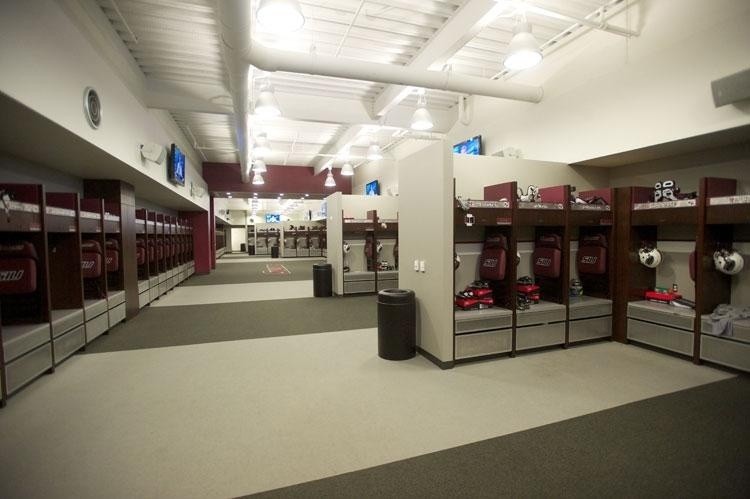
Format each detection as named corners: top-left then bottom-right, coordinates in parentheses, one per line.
top-left (241, 244), bottom-right (246, 252)
top-left (313, 263), bottom-right (332, 297)
top-left (271, 247), bottom-right (278, 258)
top-left (376, 288), bottom-right (416, 360)
top-left (249, 245), bottom-right (255, 255)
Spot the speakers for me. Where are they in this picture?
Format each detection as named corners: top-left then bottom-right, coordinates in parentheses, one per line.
top-left (711, 67), bottom-right (750, 108)
top-left (490, 146), bottom-right (516, 158)
top-left (141, 142), bottom-right (167, 165)
top-left (192, 183), bottom-right (208, 198)
top-left (221, 208), bottom-right (226, 216)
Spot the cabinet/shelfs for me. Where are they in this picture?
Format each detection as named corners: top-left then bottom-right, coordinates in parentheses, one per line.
top-left (0, 182), bottom-right (195, 408)
top-left (454, 176), bottom-right (622, 364)
top-left (623, 175), bottom-right (748, 373)
top-left (245, 223), bottom-right (327, 258)
top-left (342, 208), bottom-right (398, 295)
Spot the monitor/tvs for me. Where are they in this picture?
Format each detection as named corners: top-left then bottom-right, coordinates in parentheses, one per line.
top-left (266, 214), bottom-right (280, 223)
top-left (453, 134), bottom-right (482, 155)
top-left (171, 143), bottom-right (186, 187)
top-left (366, 179), bottom-right (379, 195)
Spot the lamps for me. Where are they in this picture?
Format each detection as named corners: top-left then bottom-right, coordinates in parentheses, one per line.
top-left (411, 93), bottom-right (432, 132)
top-left (323, 159), bottom-right (355, 187)
top-left (137, 142), bottom-right (166, 165)
top-left (500, 12), bottom-right (544, 72)
top-left (366, 143), bottom-right (382, 160)
top-left (254, 0), bottom-right (305, 33)
top-left (250, 159), bottom-right (268, 185)
top-left (190, 181), bottom-right (207, 197)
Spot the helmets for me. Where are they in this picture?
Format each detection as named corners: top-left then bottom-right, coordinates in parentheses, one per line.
top-left (639, 247), bottom-right (663, 269)
top-left (713, 249), bottom-right (745, 275)
